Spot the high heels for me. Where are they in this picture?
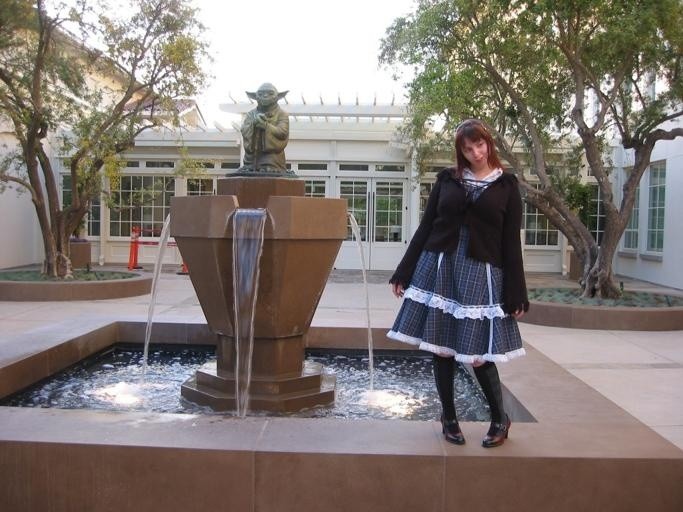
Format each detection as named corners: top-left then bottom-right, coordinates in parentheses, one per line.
top-left (483, 414), bottom-right (511, 447)
top-left (441, 414), bottom-right (465, 445)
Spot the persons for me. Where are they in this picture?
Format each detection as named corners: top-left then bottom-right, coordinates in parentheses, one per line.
top-left (386, 118), bottom-right (530, 448)
top-left (237, 83), bottom-right (290, 168)
